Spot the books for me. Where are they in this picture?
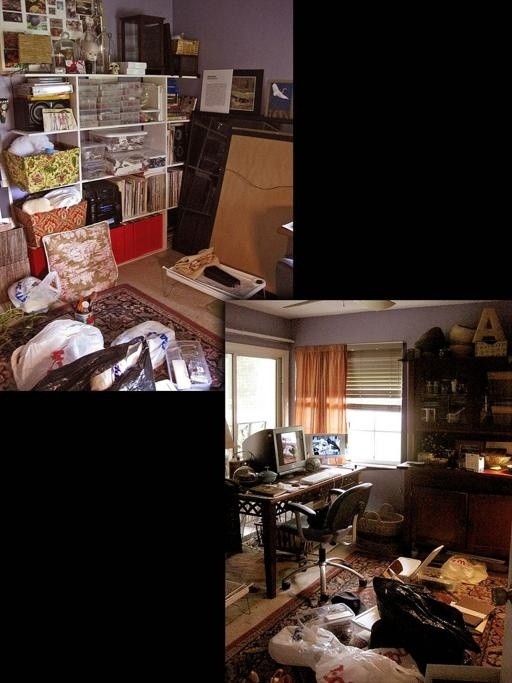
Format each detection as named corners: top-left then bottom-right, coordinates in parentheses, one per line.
top-left (250, 483), bottom-right (286, 497)
top-left (165, 79), bottom-right (198, 165)
top-left (14, 77), bottom-right (72, 97)
top-left (453, 595), bottom-right (496, 633)
top-left (113, 167), bottom-right (185, 222)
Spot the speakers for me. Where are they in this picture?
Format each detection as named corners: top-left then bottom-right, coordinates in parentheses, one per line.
top-left (306, 458), bottom-right (320, 472)
top-left (13, 96), bottom-right (72, 132)
top-left (175, 125), bottom-right (187, 162)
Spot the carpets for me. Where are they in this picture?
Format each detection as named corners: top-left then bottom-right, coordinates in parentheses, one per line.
top-left (224, 514), bottom-right (357, 626)
top-left (224, 533), bottom-right (512, 682)
top-left (0, 279), bottom-right (227, 392)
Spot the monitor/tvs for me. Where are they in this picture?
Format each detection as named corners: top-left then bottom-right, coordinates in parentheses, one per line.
top-left (306, 433), bottom-right (349, 458)
top-left (268, 426), bottom-right (308, 477)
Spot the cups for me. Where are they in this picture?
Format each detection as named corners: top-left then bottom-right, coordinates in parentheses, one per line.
top-left (76, 309), bottom-right (95, 327)
top-left (424, 408), bottom-right (435, 423)
top-left (445, 413), bottom-right (460, 423)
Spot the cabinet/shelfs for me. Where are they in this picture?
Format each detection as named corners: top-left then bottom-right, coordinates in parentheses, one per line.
top-left (0, 69), bottom-right (203, 303)
top-left (396, 353), bottom-right (511, 572)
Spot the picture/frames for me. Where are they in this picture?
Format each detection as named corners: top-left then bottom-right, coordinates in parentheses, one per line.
top-left (229, 68), bottom-right (294, 119)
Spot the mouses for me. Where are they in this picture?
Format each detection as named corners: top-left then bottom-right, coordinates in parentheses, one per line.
top-left (292, 483), bottom-right (299, 487)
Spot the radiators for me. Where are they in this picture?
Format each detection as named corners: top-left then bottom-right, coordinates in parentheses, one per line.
top-left (161, 257), bottom-right (268, 317)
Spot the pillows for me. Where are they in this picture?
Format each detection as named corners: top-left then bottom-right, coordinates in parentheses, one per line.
top-left (40, 220), bottom-right (121, 299)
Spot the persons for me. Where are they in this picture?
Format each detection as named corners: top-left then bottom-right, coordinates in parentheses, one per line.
top-left (81, 41), bottom-right (100, 62)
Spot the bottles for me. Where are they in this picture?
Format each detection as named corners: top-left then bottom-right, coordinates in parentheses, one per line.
top-left (425, 378), bottom-right (464, 394)
top-left (479, 386), bottom-right (492, 427)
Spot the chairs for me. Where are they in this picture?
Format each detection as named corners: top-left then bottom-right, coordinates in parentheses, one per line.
top-left (284, 480), bottom-right (374, 600)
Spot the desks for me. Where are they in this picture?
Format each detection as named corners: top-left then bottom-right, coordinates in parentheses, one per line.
top-left (224, 460), bottom-right (368, 598)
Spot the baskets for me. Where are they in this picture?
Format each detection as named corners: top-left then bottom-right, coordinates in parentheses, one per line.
top-left (358, 504), bottom-right (404, 536)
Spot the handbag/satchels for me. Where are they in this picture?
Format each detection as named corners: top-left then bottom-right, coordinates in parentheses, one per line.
top-left (174, 246), bottom-right (219, 279)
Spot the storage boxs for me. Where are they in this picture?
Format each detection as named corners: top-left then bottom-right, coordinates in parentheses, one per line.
top-left (6, 194), bottom-right (88, 250)
top-left (2, 138), bottom-right (79, 195)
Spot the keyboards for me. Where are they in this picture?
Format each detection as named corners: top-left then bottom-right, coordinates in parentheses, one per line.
top-left (301, 466), bottom-right (342, 485)
